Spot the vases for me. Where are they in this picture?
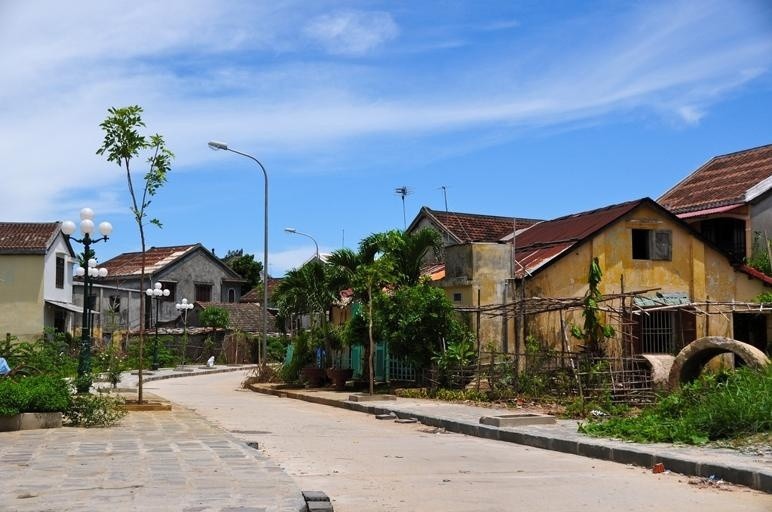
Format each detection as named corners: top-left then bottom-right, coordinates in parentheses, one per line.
top-left (301, 366), bottom-right (325, 387)
top-left (335, 369), bottom-right (348, 386)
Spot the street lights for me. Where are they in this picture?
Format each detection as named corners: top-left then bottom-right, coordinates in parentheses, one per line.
top-left (59, 206), bottom-right (114, 394)
top-left (175, 298), bottom-right (194, 371)
top-left (282, 227), bottom-right (320, 259)
top-left (145, 281), bottom-right (171, 371)
top-left (207, 139), bottom-right (269, 367)
top-left (76, 259), bottom-right (108, 387)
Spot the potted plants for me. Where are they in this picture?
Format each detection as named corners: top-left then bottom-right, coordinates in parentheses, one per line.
top-left (0, 370), bottom-right (71, 431)
top-left (431, 337), bottom-right (475, 389)
